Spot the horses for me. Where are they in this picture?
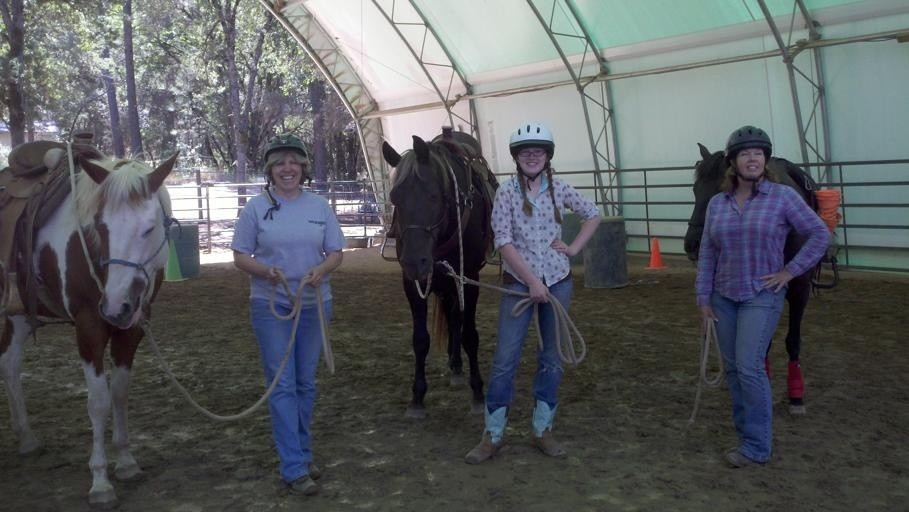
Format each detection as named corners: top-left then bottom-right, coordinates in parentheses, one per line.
top-left (683, 142), bottom-right (822, 415)
top-left (381, 131), bottom-right (500, 420)
top-left (0, 149), bottom-right (180, 512)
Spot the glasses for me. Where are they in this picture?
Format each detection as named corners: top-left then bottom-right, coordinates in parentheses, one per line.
top-left (518, 147), bottom-right (546, 158)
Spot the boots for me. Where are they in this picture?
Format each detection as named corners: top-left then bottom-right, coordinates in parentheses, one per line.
top-left (465, 404), bottom-right (510, 466)
top-left (531, 398), bottom-right (567, 458)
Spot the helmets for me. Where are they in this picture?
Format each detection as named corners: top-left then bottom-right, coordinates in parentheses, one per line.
top-left (725, 126), bottom-right (772, 157)
top-left (264, 132), bottom-right (307, 157)
top-left (510, 121), bottom-right (554, 147)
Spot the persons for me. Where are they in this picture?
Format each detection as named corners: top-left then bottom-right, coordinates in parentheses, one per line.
top-left (230, 133), bottom-right (347, 497)
top-left (465, 120), bottom-right (602, 466)
top-left (694, 126), bottom-right (833, 468)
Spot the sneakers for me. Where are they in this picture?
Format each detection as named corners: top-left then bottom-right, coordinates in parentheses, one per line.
top-left (727, 452), bottom-right (766, 468)
top-left (289, 476), bottom-right (318, 496)
top-left (307, 462), bottom-right (323, 478)
